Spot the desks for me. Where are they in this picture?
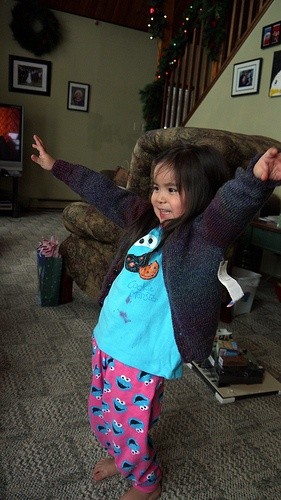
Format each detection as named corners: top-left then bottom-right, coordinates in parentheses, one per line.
top-left (250, 216), bottom-right (281, 287)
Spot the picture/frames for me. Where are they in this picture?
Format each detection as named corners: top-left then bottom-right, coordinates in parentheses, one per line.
top-left (67, 80), bottom-right (91, 112)
top-left (261, 20), bottom-right (281, 50)
top-left (231, 58), bottom-right (263, 97)
top-left (7, 55), bottom-right (52, 97)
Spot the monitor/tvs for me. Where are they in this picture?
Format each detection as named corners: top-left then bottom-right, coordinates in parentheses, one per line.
top-left (0, 103), bottom-right (24, 175)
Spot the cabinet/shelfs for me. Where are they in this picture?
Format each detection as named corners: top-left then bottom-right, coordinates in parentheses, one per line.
top-left (0, 168), bottom-right (24, 218)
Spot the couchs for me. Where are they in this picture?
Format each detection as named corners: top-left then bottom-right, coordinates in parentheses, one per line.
top-left (57, 126), bottom-right (281, 322)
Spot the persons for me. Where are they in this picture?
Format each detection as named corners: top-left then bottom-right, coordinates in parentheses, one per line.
top-left (30, 135), bottom-right (281, 500)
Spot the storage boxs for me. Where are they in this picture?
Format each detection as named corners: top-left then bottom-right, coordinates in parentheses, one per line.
top-left (232, 267), bottom-right (261, 317)
